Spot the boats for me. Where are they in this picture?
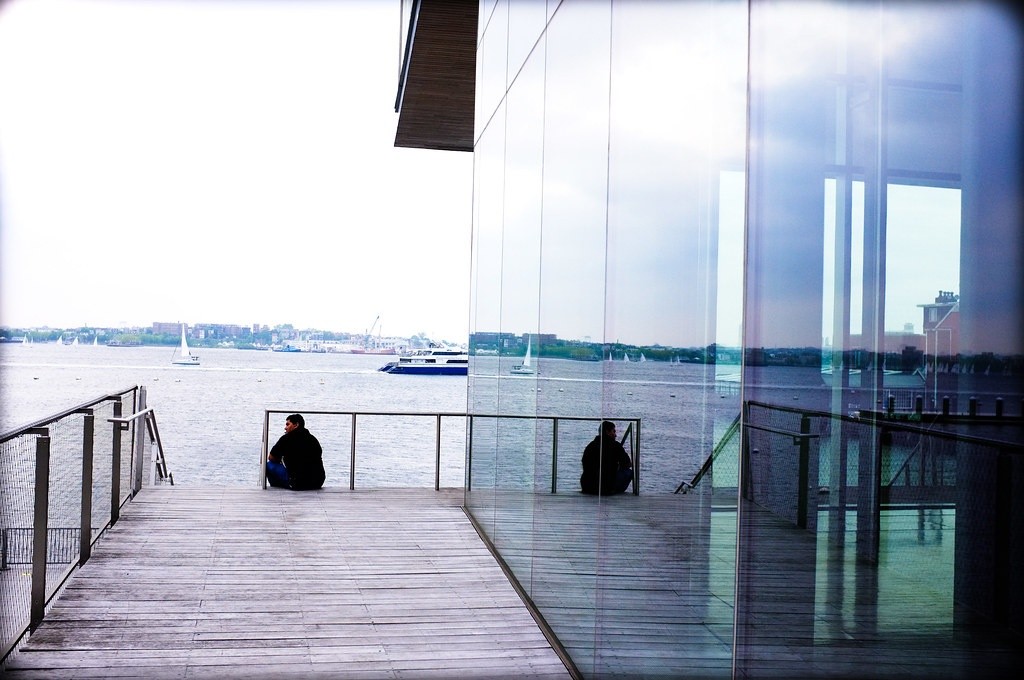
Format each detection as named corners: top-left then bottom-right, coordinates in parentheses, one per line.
top-left (377, 339), bottom-right (467, 376)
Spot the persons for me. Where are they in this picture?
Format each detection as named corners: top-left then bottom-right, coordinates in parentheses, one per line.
top-left (579, 420), bottom-right (633, 497)
top-left (267, 414), bottom-right (326, 491)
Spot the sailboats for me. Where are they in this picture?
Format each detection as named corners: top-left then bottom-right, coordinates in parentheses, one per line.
top-left (71, 336), bottom-right (78, 349)
top-left (55, 335), bottom-right (62, 347)
top-left (609, 353), bottom-right (613, 364)
top-left (94, 336), bottom-right (98, 348)
top-left (510, 334), bottom-right (534, 374)
top-left (30, 337), bottom-right (35, 348)
top-left (670, 355), bottom-right (673, 367)
top-left (21, 334), bottom-right (28, 348)
top-left (170, 321), bottom-right (201, 365)
top-left (624, 352), bottom-right (629, 365)
top-left (641, 353), bottom-right (646, 365)
top-left (675, 355), bottom-right (683, 366)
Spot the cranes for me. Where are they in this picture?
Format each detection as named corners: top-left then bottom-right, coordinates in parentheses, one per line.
top-left (365, 316), bottom-right (379, 343)
